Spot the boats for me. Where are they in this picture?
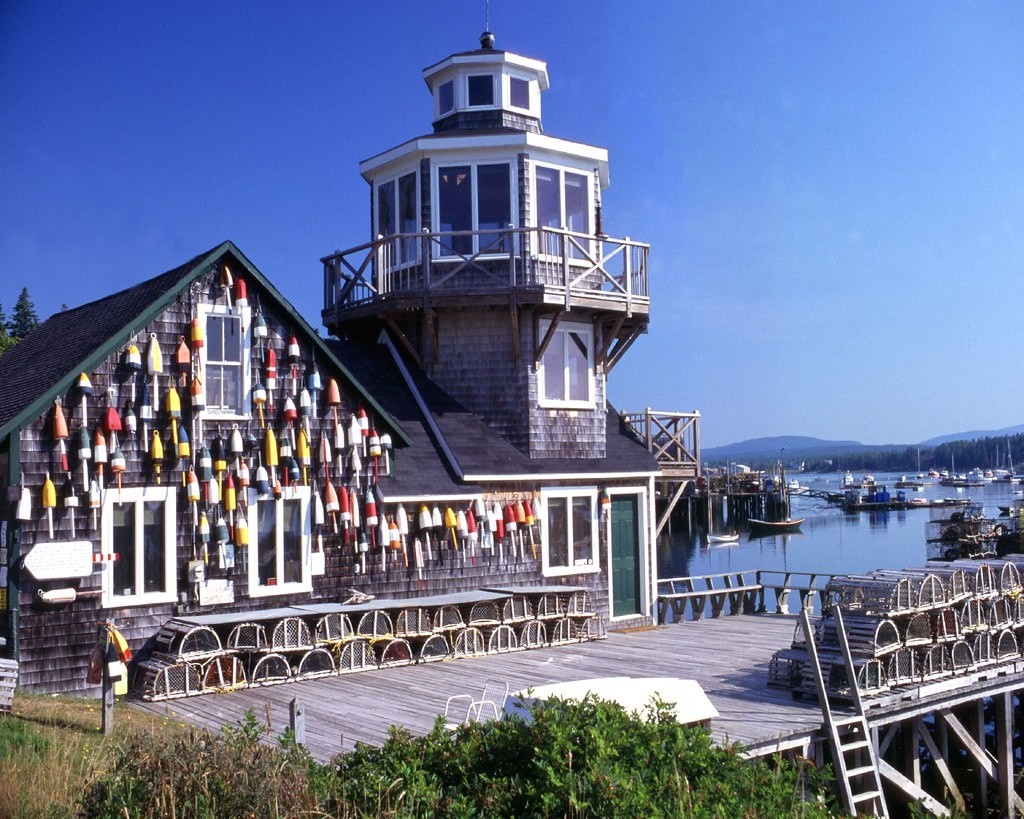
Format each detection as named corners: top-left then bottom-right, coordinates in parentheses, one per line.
top-left (706, 534), bottom-right (740, 544)
top-left (769, 439), bottom-right (1024, 517)
top-left (747, 518), bottom-right (806, 532)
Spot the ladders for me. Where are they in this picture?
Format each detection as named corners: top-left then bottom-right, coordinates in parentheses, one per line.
top-left (797, 606), bottom-right (892, 819)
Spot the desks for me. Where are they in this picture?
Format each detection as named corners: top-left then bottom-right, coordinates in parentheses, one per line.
top-left (512, 678), bottom-right (722, 736)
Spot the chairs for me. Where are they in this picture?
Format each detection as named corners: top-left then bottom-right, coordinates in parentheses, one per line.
top-left (443, 677), bottom-right (507, 731)
